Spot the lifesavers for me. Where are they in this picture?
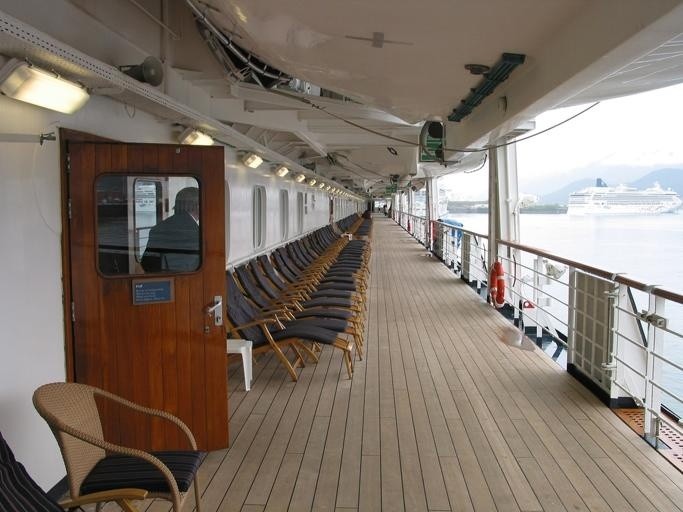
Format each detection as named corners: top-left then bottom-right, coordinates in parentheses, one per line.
top-left (489, 261), bottom-right (505, 308)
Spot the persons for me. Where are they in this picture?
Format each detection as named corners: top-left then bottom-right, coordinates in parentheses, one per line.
top-left (138, 187), bottom-right (201, 273)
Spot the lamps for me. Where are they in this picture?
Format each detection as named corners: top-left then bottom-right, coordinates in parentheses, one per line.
top-left (241, 151), bottom-right (317, 187)
top-left (176, 127), bottom-right (214, 146)
top-left (0, 56), bottom-right (90, 116)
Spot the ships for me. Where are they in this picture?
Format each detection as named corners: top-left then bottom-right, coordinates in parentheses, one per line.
top-left (565, 185), bottom-right (680, 216)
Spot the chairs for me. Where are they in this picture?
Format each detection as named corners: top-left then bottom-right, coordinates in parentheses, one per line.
top-left (31, 377), bottom-right (210, 510)
top-left (225, 210), bottom-right (373, 390)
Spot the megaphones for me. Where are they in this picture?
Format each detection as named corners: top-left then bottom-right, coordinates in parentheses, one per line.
top-left (125, 55), bottom-right (164, 85)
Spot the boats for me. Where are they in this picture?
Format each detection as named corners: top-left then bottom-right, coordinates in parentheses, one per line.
top-left (399, 187), bottom-right (448, 224)
